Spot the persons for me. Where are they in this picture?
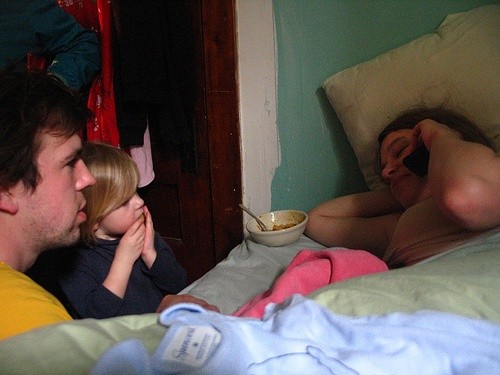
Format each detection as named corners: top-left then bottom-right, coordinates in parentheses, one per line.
top-left (0, 69), bottom-right (221, 342)
top-left (56, 140), bottom-right (189, 320)
top-left (0, 0), bottom-right (104, 94)
top-left (305, 108), bottom-right (500, 271)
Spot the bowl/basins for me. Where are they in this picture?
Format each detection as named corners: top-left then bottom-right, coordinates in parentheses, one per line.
top-left (246, 210), bottom-right (309, 247)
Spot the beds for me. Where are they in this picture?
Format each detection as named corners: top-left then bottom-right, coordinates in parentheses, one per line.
top-left (0, 232), bottom-right (498, 374)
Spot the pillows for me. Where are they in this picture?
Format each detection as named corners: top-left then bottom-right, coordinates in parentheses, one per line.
top-left (320, 4), bottom-right (499, 192)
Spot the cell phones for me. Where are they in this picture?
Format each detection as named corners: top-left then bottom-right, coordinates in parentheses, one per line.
top-left (402, 142), bottom-right (430, 178)
